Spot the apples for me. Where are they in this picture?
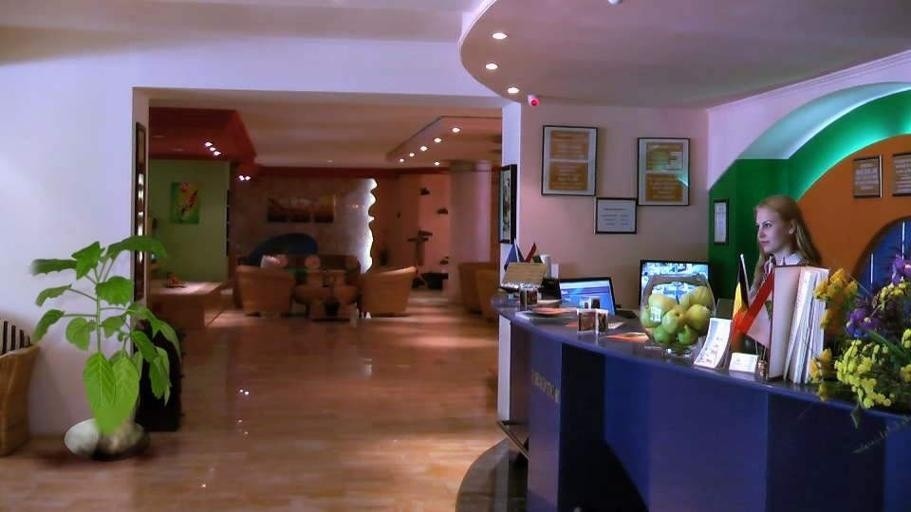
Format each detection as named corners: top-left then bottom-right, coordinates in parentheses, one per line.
top-left (640, 285), bottom-right (715, 347)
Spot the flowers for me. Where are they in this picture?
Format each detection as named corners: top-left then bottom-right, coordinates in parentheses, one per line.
top-left (805, 252), bottom-right (911, 411)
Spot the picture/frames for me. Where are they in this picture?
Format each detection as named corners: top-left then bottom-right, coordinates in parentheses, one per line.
top-left (496, 160), bottom-right (518, 245)
top-left (712, 197), bottom-right (732, 249)
top-left (539, 121), bottom-right (600, 199)
top-left (634, 134), bottom-right (691, 209)
top-left (593, 195), bottom-right (637, 237)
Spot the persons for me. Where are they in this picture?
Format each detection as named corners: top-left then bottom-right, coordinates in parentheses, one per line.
top-left (748, 194), bottom-right (822, 304)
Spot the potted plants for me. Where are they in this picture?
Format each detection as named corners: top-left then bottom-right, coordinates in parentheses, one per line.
top-left (24, 233), bottom-right (181, 462)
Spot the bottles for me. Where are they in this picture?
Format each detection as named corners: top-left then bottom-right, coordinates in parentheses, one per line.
top-left (540, 254), bottom-right (551, 277)
top-left (519, 282), bottom-right (537, 308)
top-left (755, 360), bottom-right (770, 385)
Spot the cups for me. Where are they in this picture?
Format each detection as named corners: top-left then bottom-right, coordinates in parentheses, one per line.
top-left (640, 272), bottom-right (718, 359)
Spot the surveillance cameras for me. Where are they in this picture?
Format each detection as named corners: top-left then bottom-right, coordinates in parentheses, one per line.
top-left (527, 94), bottom-right (542, 107)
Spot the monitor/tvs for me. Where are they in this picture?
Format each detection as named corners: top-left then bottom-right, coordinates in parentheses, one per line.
top-left (559, 277), bottom-right (616, 316)
top-left (639, 259), bottom-right (709, 306)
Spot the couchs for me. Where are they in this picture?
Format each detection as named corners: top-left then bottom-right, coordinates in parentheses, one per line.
top-left (0, 319), bottom-right (33, 456)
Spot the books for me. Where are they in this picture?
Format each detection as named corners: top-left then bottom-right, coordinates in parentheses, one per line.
top-left (767, 261), bottom-right (832, 384)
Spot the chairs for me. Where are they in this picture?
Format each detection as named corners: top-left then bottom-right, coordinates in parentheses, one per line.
top-left (233, 246), bottom-right (500, 327)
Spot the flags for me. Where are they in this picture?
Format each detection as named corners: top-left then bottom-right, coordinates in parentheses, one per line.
top-left (734, 254), bottom-right (749, 342)
top-left (741, 269), bottom-right (776, 349)
top-left (504, 239), bottom-right (524, 275)
top-left (527, 243), bottom-right (543, 265)
